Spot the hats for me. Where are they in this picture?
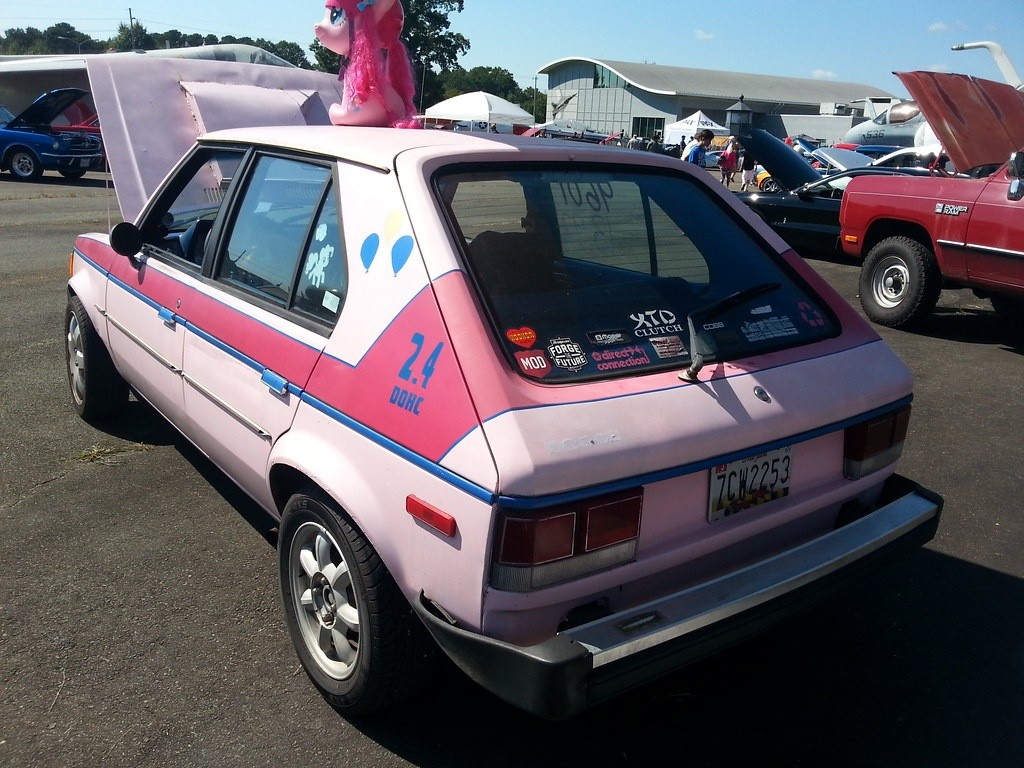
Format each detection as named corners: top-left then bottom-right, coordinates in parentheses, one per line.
top-left (812, 162), bottom-right (821, 168)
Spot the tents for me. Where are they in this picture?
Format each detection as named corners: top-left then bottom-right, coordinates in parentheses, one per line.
top-left (663, 110), bottom-right (730, 146)
top-left (424, 91), bottom-right (600, 147)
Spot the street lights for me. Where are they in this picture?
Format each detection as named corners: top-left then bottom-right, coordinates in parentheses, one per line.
top-left (57, 36), bottom-right (99, 55)
top-left (418, 54), bottom-right (428, 115)
top-left (531, 76), bottom-right (538, 137)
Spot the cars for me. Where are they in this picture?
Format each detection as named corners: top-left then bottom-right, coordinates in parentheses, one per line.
top-left (729, 37), bottom-right (1023, 246)
top-left (837, 68), bottom-right (1023, 333)
top-left (0, 87), bottom-right (104, 185)
top-left (64, 52), bottom-right (944, 722)
top-left (53, 106), bottom-right (110, 171)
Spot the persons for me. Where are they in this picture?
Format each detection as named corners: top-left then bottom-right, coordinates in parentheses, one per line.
top-left (679, 128), bottom-right (715, 170)
top-left (538, 130), bottom-right (547, 138)
top-left (717, 129), bottom-right (758, 192)
top-left (626, 134), bottom-right (665, 155)
top-left (490, 124), bottom-right (500, 134)
top-left (572, 131), bottom-right (585, 140)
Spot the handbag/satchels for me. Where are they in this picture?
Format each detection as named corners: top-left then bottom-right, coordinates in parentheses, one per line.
top-left (717, 155), bottom-right (726, 165)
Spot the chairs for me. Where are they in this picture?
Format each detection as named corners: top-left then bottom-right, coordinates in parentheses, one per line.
top-left (465, 229), bottom-right (562, 333)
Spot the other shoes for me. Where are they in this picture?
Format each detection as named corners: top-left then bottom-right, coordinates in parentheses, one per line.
top-left (740, 183), bottom-right (746, 191)
top-left (730, 177), bottom-right (735, 182)
